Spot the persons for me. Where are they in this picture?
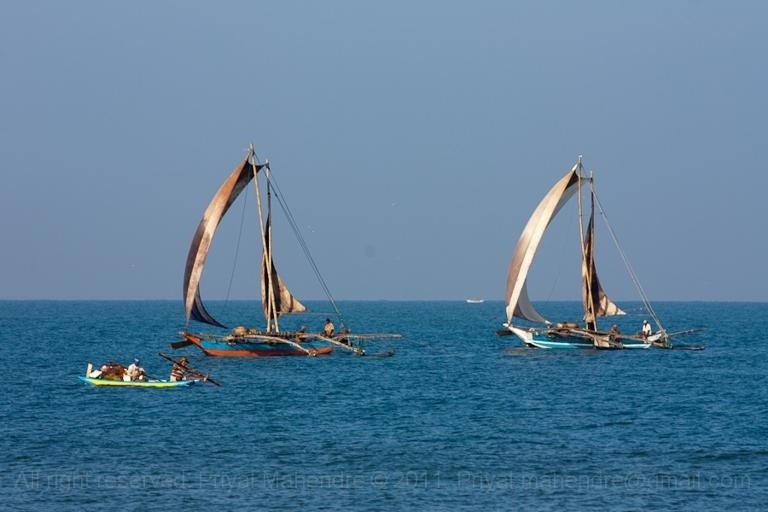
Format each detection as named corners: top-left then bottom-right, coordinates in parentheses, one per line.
top-left (126, 357), bottom-right (145, 382)
top-left (323, 319), bottom-right (334, 338)
top-left (641, 320), bottom-right (651, 343)
top-left (610, 324), bottom-right (618, 335)
top-left (170, 357), bottom-right (200, 381)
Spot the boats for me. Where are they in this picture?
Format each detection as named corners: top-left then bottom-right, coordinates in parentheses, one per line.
top-left (78, 375), bottom-right (210, 388)
top-left (466, 296), bottom-right (486, 304)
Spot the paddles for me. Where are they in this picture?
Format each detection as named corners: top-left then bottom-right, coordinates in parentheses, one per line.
top-left (158, 351), bottom-right (226, 388)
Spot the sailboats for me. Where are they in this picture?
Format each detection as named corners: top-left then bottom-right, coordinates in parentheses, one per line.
top-left (170, 145), bottom-right (405, 357)
top-left (496, 154), bottom-right (705, 352)
